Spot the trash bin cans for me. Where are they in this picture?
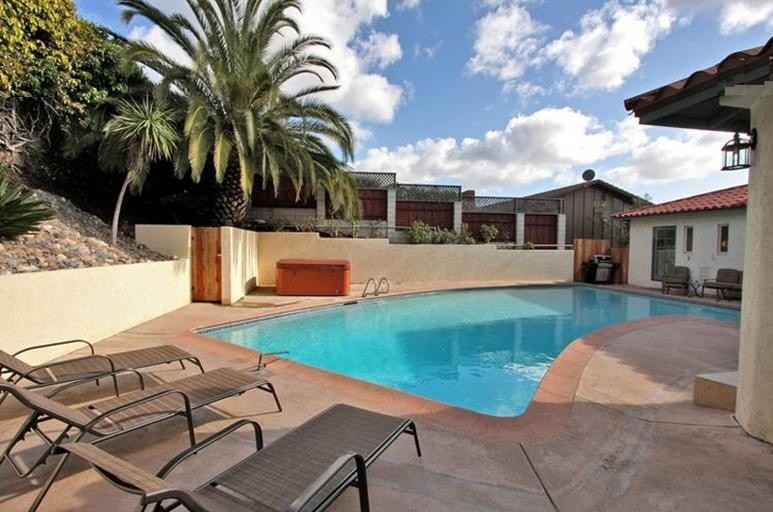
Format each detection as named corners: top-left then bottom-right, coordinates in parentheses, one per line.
top-left (594, 255), bottom-right (615, 284)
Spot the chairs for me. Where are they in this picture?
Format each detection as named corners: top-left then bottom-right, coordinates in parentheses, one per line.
top-left (661, 266), bottom-right (740, 302)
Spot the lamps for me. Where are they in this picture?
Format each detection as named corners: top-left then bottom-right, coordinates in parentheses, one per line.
top-left (719, 127), bottom-right (758, 171)
